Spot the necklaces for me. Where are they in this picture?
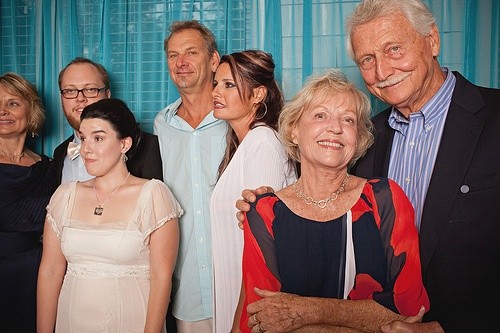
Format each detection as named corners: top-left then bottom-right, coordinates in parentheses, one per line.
top-left (292, 172), bottom-right (351, 209)
top-left (0, 146), bottom-right (27, 159)
top-left (92, 172), bottom-right (131, 216)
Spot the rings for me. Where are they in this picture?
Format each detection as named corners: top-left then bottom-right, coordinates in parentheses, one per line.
top-left (253, 313), bottom-right (258, 322)
top-left (258, 324), bottom-right (265, 332)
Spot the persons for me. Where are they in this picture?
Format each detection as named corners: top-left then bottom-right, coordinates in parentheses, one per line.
top-left (54, 56), bottom-right (163, 191)
top-left (210, 49), bottom-right (301, 333)
top-left (153, 21), bottom-right (228, 333)
top-left (235, 0), bottom-right (500, 333)
top-left (37, 98), bottom-right (184, 333)
top-left (0, 73), bottom-right (61, 333)
top-left (239, 69), bottom-right (430, 333)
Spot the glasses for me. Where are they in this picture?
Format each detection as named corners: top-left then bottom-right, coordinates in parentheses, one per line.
top-left (59, 87), bottom-right (107, 99)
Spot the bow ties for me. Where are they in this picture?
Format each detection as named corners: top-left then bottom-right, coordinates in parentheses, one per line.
top-left (67, 141), bottom-right (81, 161)
